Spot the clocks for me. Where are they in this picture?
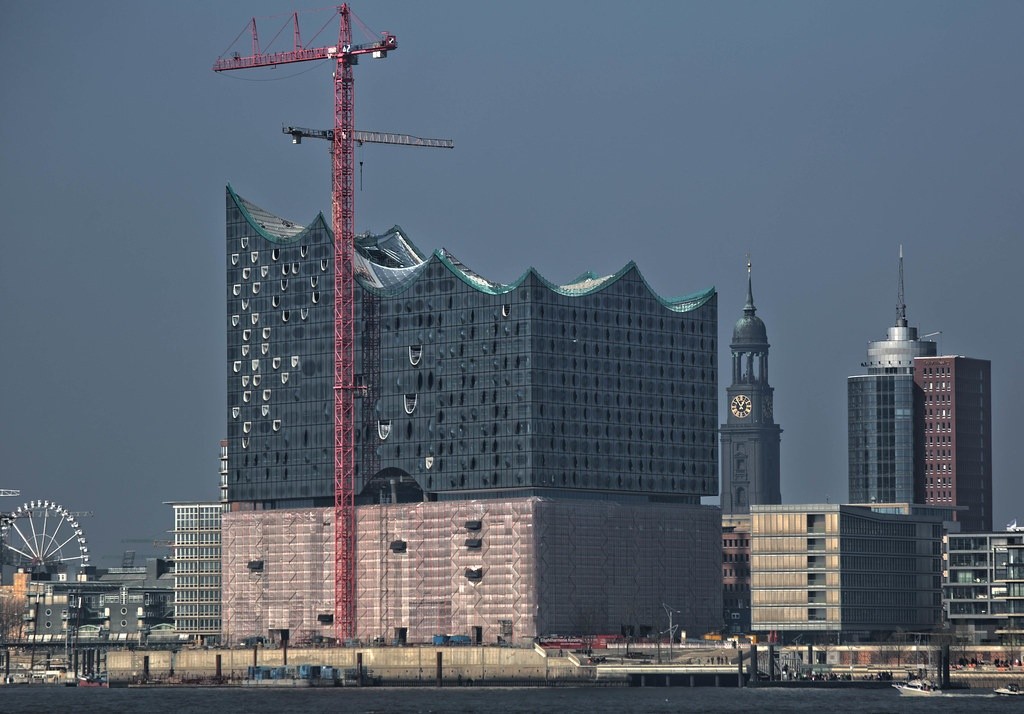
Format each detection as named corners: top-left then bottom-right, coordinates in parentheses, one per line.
top-left (730, 395), bottom-right (752, 417)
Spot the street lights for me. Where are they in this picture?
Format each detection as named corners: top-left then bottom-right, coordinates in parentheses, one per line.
top-left (660, 603), bottom-right (681, 662)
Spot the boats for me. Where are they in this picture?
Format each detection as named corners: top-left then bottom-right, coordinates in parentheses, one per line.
top-left (993, 683), bottom-right (1024, 696)
top-left (891, 677), bottom-right (943, 696)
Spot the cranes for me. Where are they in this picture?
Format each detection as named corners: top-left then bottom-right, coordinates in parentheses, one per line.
top-left (280, 123), bottom-right (455, 232)
top-left (208, 1), bottom-right (401, 648)
top-left (102, 526), bottom-right (176, 561)
top-left (0, 508), bottom-right (96, 586)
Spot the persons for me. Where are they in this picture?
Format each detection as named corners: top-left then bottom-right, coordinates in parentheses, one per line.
top-left (906, 668), bottom-right (928, 681)
top-left (788, 670), bottom-right (851, 680)
top-left (923, 674), bottom-right (936, 691)
top-left (710, 655), bottom-right (728, 666)
top-left (863, 670), bottom-right (894, 681)
